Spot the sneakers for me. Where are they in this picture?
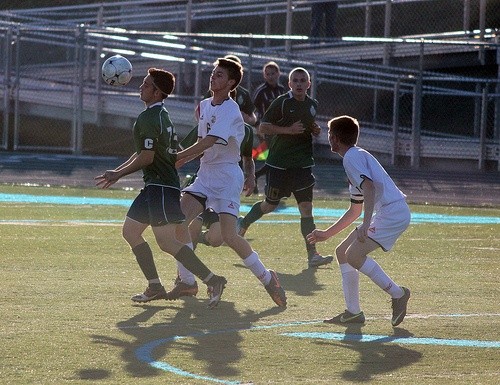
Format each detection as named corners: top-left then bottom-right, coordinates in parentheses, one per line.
top-left (323, 310), bottom-right (365, 323)
top-left (173, 276), bottom-right (181, 287)
top-left (391, 286), bottom-right (410, 326)
top-left (165, 281), bottom-right (198, 301)
top-left (207, 276), bottom-right (227, 307)
top-left (239, 216), bottom-right (247, 237)
top-left (131, 286), bottom-right (167, 303)
top-left (264, 270), bottom-right (287, 308)
top-left (308, 252), bottom-right (333, 267)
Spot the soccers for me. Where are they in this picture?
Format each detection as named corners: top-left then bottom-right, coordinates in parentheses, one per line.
top-left (102, 55), bottom-right (132, 86)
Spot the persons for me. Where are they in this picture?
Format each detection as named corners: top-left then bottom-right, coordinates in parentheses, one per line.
top-left (196, 56), bottom-right (283, 195)
top-left (305, 115), bottom-right (414, 326)
top-left (96, 68), bottom-right (227, 309)
top-left (169, 58), bottom-right (287, 308)
top-left (237, 67), bottom-right (334, 267)
